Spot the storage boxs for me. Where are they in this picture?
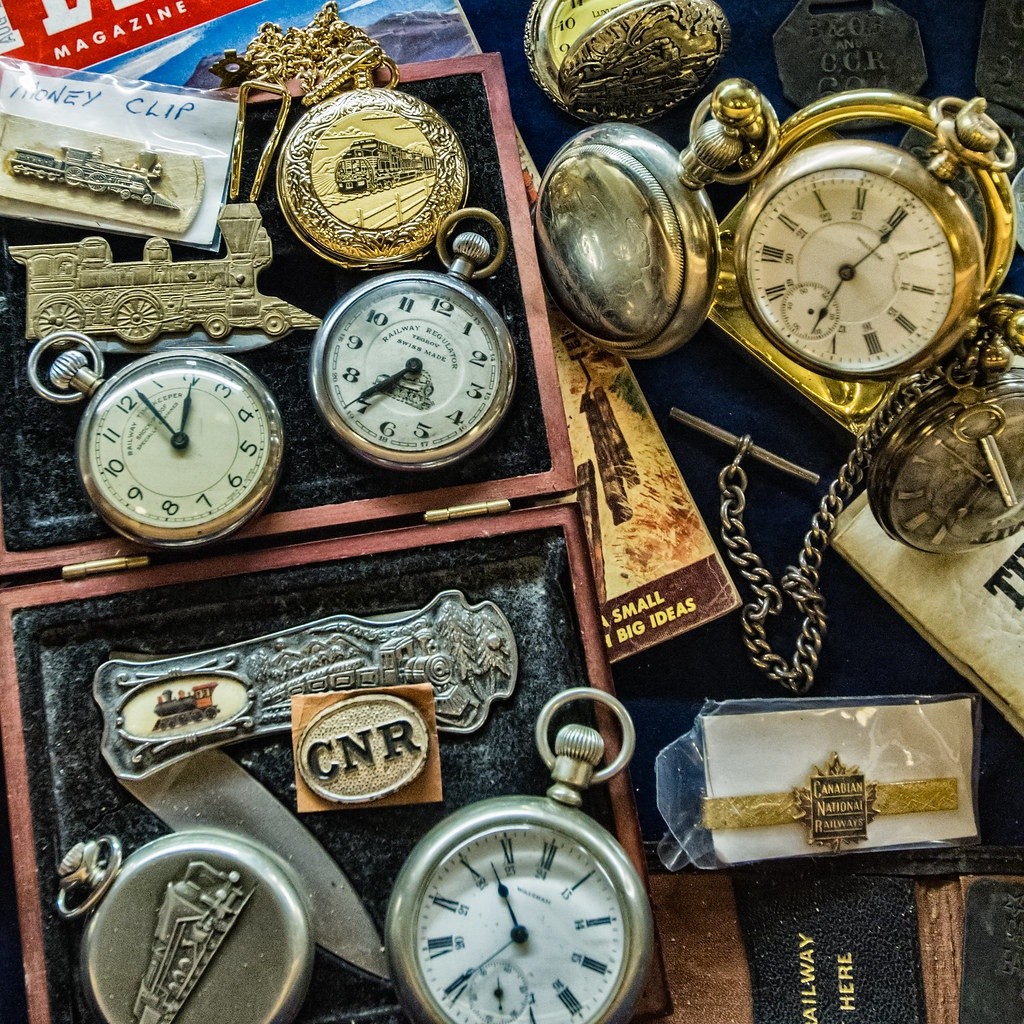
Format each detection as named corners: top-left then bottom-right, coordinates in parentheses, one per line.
top-left (0, 52), bottom-right (679, 1024)
